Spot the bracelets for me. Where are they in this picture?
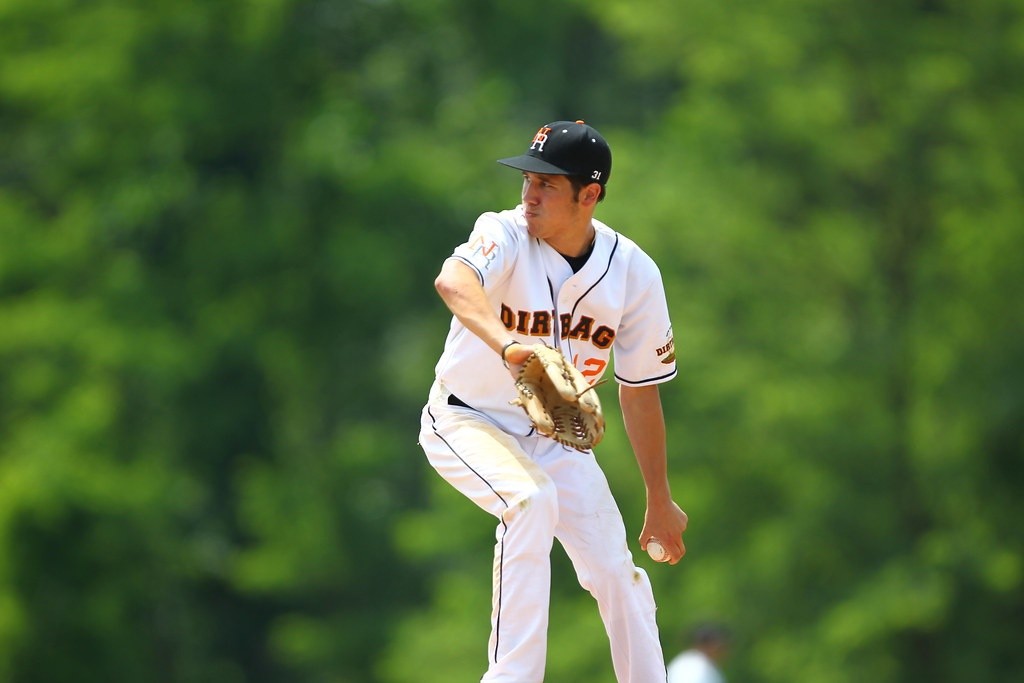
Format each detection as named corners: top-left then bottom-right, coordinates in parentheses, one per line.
top-left (502, 340), bottom-right (521, 369)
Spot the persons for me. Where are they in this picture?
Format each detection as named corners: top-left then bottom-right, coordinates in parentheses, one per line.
top-left (666, 621), bottom-right (730, 683)
top-left (419, 120), bottom-right (689, 683)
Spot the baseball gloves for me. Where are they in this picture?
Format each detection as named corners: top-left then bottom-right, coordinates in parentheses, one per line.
top-left (514, 344), bottom-right (605, 450)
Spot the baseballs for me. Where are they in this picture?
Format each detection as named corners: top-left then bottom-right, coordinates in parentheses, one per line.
top-left (646, 535), bottom-right (671, 563)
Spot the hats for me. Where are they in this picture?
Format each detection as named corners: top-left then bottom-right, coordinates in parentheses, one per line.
top-left (497, 119), bottom-right (611, 185)
